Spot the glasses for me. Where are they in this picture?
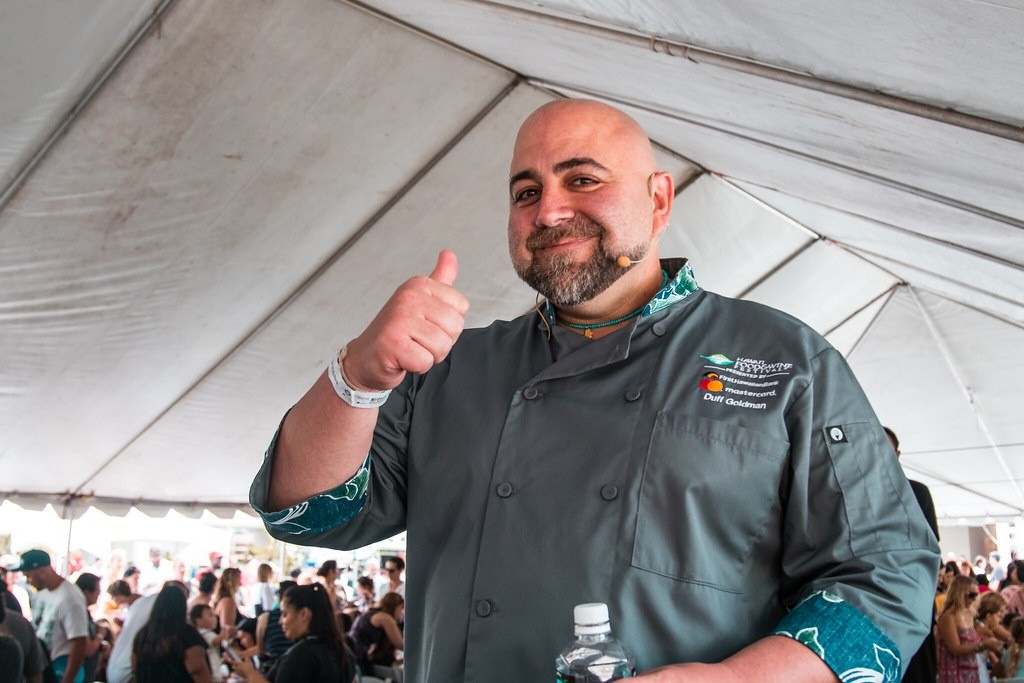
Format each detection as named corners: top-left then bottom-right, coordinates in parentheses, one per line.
top-left (965, 592), bottom-right (978, 601)
top-left (384, 568), bottom-right (395, 574)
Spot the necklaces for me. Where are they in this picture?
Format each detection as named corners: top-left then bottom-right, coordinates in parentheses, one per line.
top-left (558, 270), bottom-right (668, 338)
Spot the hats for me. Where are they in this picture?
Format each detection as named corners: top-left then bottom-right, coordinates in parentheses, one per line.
top-left (11, 549), bottom-right (50, 572)
top-left (210, 552), bottom-right (222, 560)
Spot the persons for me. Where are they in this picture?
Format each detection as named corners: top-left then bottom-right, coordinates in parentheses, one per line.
top-left (248, 99), bottom-right (942, 683)
top-left (882, 425), bottom-right (942, 555)
top-left (1, 546), bottom-right (1024, 682)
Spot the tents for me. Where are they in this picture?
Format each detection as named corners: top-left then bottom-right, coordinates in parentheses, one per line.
top-left (2, 2), bottom-right (1020, 533)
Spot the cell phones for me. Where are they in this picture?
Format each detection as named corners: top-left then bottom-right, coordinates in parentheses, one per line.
top-left (224, 641), bottom-right (242, 663)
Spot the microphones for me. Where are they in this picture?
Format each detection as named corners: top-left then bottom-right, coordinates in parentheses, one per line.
top-left (618, 232), bottom-right (666, 267)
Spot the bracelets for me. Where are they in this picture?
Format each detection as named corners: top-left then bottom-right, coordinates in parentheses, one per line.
top-left (325, 344), bottom-right (392, 407)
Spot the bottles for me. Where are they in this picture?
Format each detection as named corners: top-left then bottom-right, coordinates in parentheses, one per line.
top-left (555, 602), bottom-right (636, 683)
